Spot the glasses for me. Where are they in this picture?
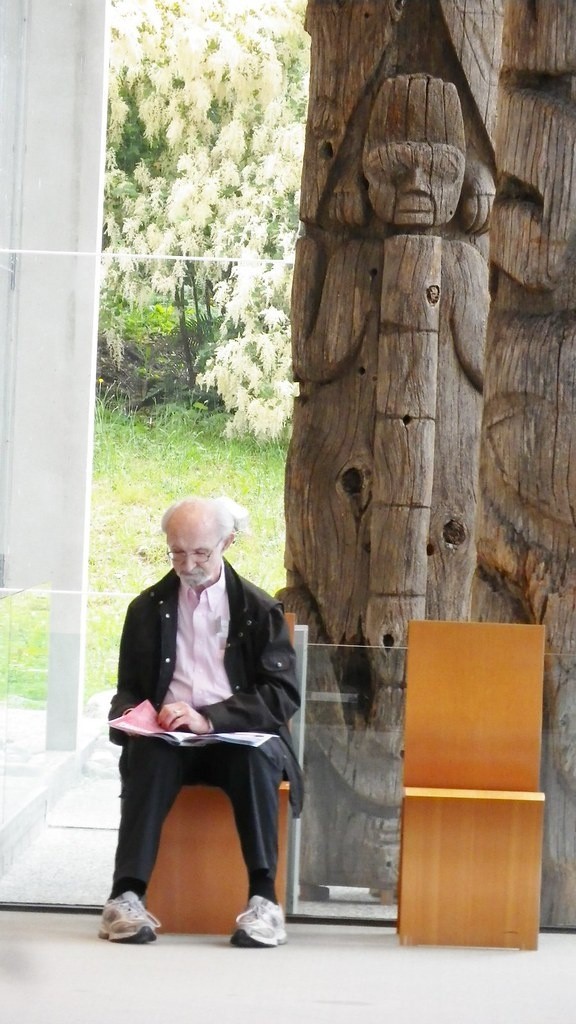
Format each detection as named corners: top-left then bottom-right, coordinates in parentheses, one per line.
top-left (167, 539), bottom-right (222, 563)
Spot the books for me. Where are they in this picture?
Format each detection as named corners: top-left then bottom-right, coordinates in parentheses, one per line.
top-left (108, 700), bottom-right (277, 747)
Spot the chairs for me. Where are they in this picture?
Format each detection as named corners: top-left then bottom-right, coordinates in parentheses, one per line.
top-left (146, 613), bottom-right (299, 935)
top-left (402, 620), bottom-right (547, 949)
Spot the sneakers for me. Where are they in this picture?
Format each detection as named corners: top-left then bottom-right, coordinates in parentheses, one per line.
top-left (98, 891), bottom-right (161, 944)
top-left (230, 894), bottom-right (287, 948)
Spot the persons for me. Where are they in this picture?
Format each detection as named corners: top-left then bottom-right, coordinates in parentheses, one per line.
top-left (97, 496), bottom-right (304, 947)
